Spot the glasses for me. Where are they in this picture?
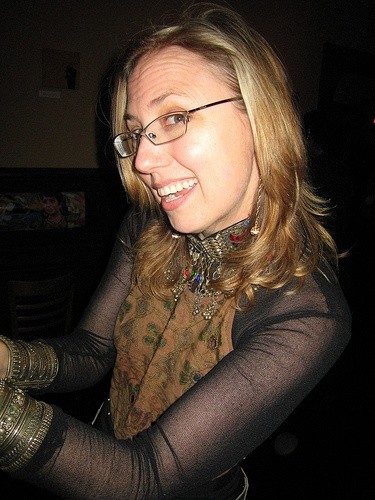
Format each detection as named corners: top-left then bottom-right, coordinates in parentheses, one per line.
top-left (107, 95), bottom-right (248, 159)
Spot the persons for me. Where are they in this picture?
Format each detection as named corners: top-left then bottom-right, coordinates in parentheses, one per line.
top-left (0, 0), bottom-right (355, 500)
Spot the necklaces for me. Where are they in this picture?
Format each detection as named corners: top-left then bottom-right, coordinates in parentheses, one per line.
top-left (165, 215), bottom-right (255, 316)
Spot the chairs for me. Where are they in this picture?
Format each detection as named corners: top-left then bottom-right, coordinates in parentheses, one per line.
top-left (7, 271), bottom-right (80, 420)
